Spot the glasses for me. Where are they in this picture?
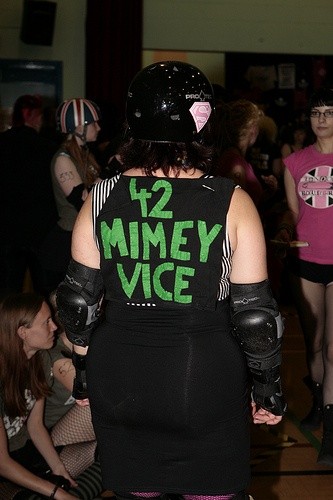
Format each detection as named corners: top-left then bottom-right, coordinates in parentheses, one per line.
top-left (310, 109), bottom-right (333, 118)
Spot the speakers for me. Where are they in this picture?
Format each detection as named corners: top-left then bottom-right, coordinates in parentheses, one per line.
top-left (21, 0), bottom-right (57, 46)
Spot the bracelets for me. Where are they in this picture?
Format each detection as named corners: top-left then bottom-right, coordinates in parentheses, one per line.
top-left (50, 484), bottom-right (58, 499)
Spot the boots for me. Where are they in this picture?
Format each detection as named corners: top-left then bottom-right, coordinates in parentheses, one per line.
top-left (316, 405), bottom-right (333, 466)
top-left (300, 382), bottom-right (323, 433)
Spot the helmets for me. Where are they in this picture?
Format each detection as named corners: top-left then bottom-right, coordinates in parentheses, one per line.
top-left (55, 96), bottom-right (101, 133)
top-left (126, 61), bottom-right (214, 143)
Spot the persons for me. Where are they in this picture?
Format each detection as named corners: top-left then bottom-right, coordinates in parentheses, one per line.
top-left (2, 93), bottom-right (56, 296)
top-left (9, 396), bottom-right (98, 500)
top-left (50, 97), bottom-right (108, 275)
top-left (280, 86), bottom-right (333, 467)
top-left (213, 53), bottom-right (333, 259)
top-left (55, 60), bottom-right (288, 500)
top-left (0, 289), bottom-right (79, 500)
